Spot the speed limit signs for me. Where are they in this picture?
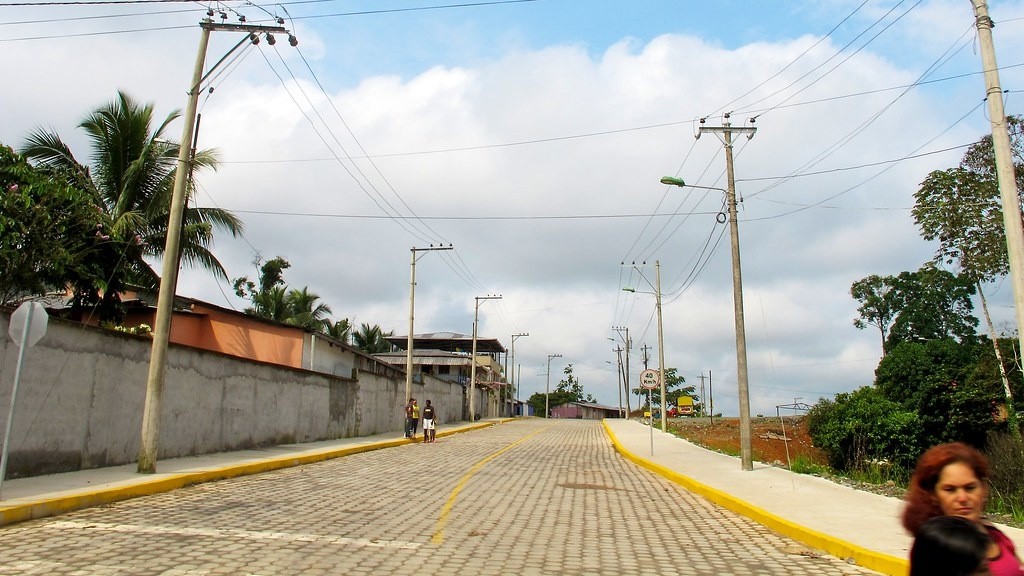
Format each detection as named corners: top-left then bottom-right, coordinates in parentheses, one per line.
top-left (640, 369), bottom-right (660, 390)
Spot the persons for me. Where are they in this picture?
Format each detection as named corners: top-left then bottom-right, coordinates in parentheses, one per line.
top-left (909, 515), bottom-right (1000, 576)
top-left (422, 399), bottom-right (435, 443)
top-left (430, 415), bottom-right (438, 443)
top-left (405, 398), bottom-right (415, 439)
top-left (410, 399), bottom-right (420, 438)
top-left (901, 441), bottom-right (1023, 576)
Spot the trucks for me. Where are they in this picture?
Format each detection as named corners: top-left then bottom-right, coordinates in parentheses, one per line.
top-left (677, 396), bottom-right (694, 418)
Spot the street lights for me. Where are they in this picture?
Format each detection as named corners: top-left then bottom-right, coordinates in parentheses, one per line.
top-left (607, 337), bottom-right (633, 420)
top-left (659, 176), bottom-right (761, 475)
top-left (622, 288), bottom-right (668, 433)
top-left (606, 360), bottom-right (622, 419)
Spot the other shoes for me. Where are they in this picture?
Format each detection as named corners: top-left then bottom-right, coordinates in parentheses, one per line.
top-left (413, 436), bottom-right (416, 439)
top-left (403, 432), bottom-right (406, 438)
